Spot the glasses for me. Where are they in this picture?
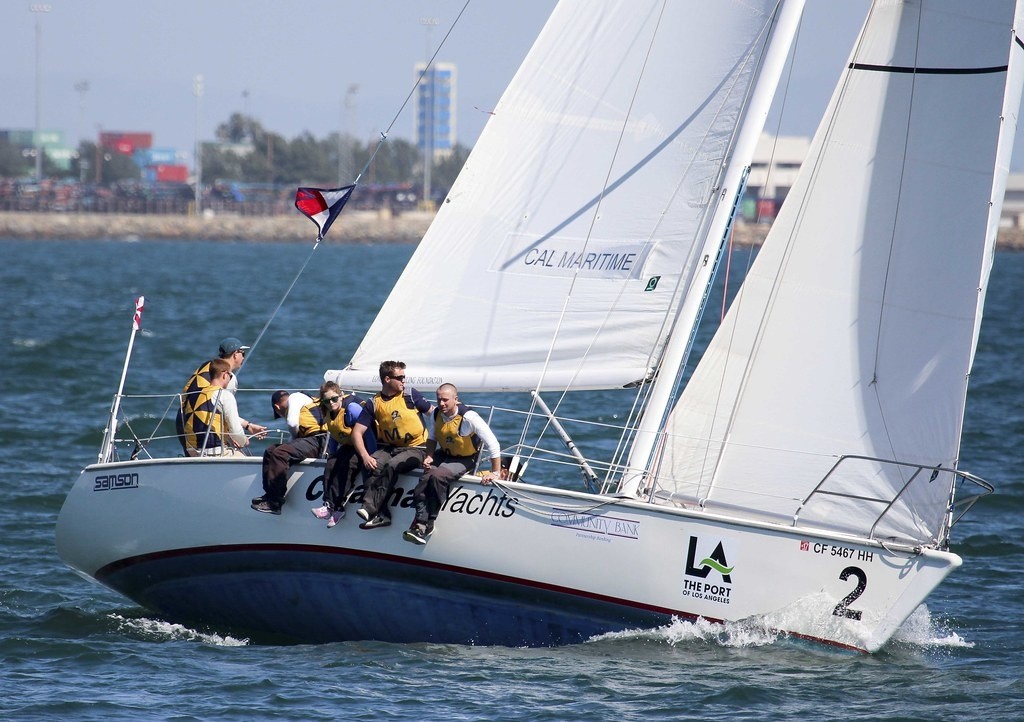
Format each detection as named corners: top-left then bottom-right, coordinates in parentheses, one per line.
top-left (389, 375), bottom-right (406, 382)
top-left (221, 371), bottom-right (233, 380)
top-left (236, 351), bottom-right (245, 357)
top-left (323, 395), bottom-right (342, 404)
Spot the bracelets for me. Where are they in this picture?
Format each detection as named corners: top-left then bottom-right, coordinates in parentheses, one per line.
top-left (245, 422), bottom-right (251, 430)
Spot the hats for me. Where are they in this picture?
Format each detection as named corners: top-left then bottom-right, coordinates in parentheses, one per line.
top-left (501, 457), bottom-right (526, 483)
top-left (219, 337), bottom-right (250, 354)
top-left (271, 390), bottom-right (290, 419)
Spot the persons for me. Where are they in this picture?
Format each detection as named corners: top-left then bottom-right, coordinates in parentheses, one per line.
top-left (175, 338), bottom-right (267, 459)
top-left (250, 360), bottom-right (501, 545)
top-left (476, 457), bottom-right (522, 482)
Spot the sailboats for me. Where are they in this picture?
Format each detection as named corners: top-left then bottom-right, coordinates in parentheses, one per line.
top-left (54, 0), bottom-right (1024, 657)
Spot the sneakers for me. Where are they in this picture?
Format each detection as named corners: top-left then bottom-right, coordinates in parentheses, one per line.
top-left (251, 501), bottom-right (281, 515)
top-left (425, 523), bottom-right (435, 537)
top-left (311, 502), bottom-right (331, 520)
top-left (252, 494), bottom-right (286, 505)
top-left (327, 511), bottom-right (345, 528)
top-left (359, 515), bottom-right (391, 530)
top-left (356, 508), bottom-right (370, 522)
top-left (402, 529), bottom-right (426, 545)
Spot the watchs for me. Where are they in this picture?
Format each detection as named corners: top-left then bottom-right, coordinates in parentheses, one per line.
top-left (492, 472), bottom-right (500, 478)
